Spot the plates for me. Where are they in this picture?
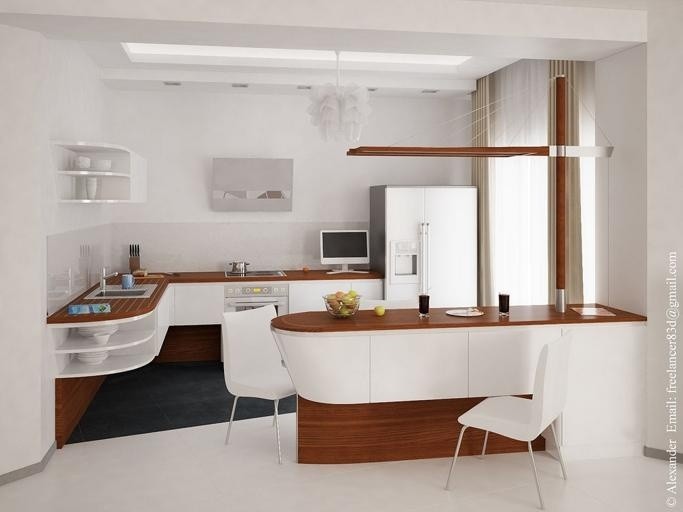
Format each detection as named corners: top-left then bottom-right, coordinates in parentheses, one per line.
top-left (446, 308), bottom-right (484, 317)
top-left (77, 326), bottom-right (117, 336)
top-left (75, 352), bottom-right (107, 365)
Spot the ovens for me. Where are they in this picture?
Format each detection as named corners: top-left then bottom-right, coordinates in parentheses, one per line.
top-left (224, 284), bottom-right (288, 318)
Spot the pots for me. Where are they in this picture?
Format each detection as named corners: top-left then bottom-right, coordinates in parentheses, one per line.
top-left (229, 261), bottom-right (249, 274)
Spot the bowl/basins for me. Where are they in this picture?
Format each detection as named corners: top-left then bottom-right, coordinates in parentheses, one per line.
top-left (95, 160), bottom-right (112, 171)
top-left (322, 294), bottom-right (361, 318)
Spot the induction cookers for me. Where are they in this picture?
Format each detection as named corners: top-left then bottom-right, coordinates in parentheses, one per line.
top-left (223, 270), bottom-right (286, 278)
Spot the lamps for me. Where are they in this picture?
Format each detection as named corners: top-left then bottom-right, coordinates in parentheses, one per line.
top-left (305, 50), bottom-right (373, 143)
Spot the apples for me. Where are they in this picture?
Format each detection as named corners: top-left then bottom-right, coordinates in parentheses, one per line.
top-left (375, 306), bottom-right (385, 316)
top-left (327, 290), bottom-right (357, 317)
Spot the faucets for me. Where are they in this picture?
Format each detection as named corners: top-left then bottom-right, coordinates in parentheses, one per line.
top-left (99, 267), bottom-right (119, 290)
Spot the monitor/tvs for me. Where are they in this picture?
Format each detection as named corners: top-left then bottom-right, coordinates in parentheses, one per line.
top-left (319, 230), bottom-right (371, 274)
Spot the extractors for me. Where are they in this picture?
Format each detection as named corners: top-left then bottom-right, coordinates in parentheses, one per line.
top-left (210, 157), bottom-right (293, 212)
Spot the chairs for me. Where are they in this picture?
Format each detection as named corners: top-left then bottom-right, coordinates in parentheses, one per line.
top-left (221, 303), bottom-right (297, 465)
top-left (444, 330), bottom-right (574, 508)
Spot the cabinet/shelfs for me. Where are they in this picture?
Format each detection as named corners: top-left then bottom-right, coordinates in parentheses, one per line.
top-left (49, 306), bottom-right (157, 380)
top-left (48, 138), bottom-right (149, 205)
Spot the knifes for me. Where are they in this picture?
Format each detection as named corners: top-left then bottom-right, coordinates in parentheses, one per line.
top-left (128, 244), bottom-right (139, 257)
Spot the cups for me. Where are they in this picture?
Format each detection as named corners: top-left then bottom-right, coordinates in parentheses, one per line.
top-left (93, 333), bottom-right (109, 346)
top-left (75, 157), bottom-right (91, 172)
top-left (86, 177), bottom-right (97, 199)
top-left (497, 291), bottom-right (510, 317)
top-left (417, 294), bottom-right (430, 319)
top-left (121, 274), bottom-right (135, 289)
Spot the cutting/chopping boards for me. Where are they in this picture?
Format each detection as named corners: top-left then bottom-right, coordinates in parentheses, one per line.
top-left (133, 274), bottom-right (164, 279)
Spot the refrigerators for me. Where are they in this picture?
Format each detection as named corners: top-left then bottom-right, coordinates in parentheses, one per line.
top-left (369, 185), bottom-right (478, 311)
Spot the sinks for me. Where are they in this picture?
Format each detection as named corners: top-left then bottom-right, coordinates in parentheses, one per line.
top-left (83, 283), bottom-right (157, 299)
